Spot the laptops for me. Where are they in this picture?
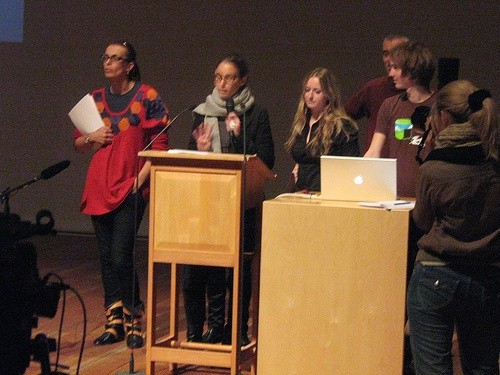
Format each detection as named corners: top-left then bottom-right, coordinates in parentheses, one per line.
top-left (320, 155), bottom-right (411, 205)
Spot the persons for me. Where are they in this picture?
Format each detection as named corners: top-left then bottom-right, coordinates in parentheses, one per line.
top-left (401, 79), bottom-right (500, 375)
top-left (284, 66), bottom-right (363, 203)
top-left (181, 51), bottom-right (277, 344)
top-left (65, 39), bottom-right (177, 349)
top-left (335, 30), bottom-right (449, 204)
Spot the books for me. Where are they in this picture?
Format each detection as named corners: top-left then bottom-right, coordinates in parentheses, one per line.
top-left (67, 92), bottom-right (106, 138)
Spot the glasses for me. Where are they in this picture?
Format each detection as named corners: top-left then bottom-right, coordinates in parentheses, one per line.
top-left (100, 54), bottom-right (133, 62)
top-left (213, 72), bottom-right (244, 82)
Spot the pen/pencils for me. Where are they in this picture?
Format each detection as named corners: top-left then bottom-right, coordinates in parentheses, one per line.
top-left (394, 202), bottom-right (411, 205)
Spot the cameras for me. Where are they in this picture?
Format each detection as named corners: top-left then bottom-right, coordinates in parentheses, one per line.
top-left (411, 105), bottom-right (433, 131)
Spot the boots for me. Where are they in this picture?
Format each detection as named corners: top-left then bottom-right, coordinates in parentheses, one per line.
top-left (93, 299), bottom-right (125, 345)
top-left (183, 287), bottom-right (206, 343)
top-left (222, 294), bottom-right (251, 345)
top-left (202, 292), bottom-right (226, 342)
top-left (122, 302), bottom-right (145, 348)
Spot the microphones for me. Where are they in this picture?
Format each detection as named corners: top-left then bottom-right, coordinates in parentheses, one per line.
top-left (33, 160), bottom-right (71, 183)
top-left (142, 104), bottom-right (199, 152)
top-left (225, 98), bottom-right (235, 143)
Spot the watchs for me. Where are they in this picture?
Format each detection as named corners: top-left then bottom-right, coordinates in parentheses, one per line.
top-left (84, 136), bottom-right (90, 146)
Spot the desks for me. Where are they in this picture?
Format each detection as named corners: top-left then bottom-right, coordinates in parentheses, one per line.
top-left (256, 191), bottom-right (416, 375)
